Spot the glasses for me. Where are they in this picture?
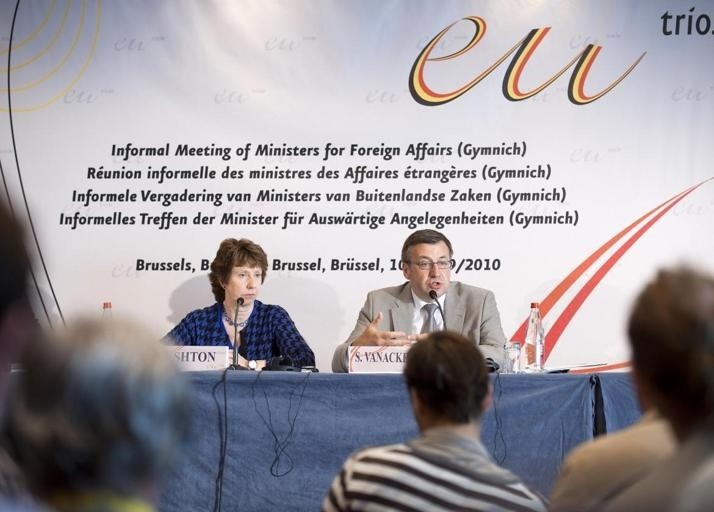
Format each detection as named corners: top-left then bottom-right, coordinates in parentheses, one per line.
top-left (408, 260), bottom-right (452, 270)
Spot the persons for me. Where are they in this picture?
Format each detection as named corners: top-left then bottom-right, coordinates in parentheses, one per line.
top-left (546, 266), bottom-right (714, 512)
top-left (155, 239), bottom-right (315, 372)
top-left (0, 191), bottom-right (176, 512)
top-left (320, 333), bottom-right (545, 512)
top-left (332, 229), bottom-right (506, 374)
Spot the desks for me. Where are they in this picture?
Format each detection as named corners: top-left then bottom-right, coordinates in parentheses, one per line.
top-left (165, 369), bottom-right (596, 511)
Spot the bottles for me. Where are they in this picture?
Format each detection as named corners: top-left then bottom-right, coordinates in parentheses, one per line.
top-left (524, 302), bottom-right (545, 375)
top-left (101, 300), bottom-right (113, 319)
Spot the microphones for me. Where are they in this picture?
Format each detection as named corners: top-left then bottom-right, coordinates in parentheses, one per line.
top-left (231, 296), bottom-right (249, 371)
top-left (427, 289), bottom-right (450, 330)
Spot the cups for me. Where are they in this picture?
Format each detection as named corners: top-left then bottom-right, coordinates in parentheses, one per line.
top-left (504, 342), bottom-right (522, 374)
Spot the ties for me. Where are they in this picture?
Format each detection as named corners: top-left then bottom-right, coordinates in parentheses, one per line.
top-left (420, 303), bottom-right (441, 334)
